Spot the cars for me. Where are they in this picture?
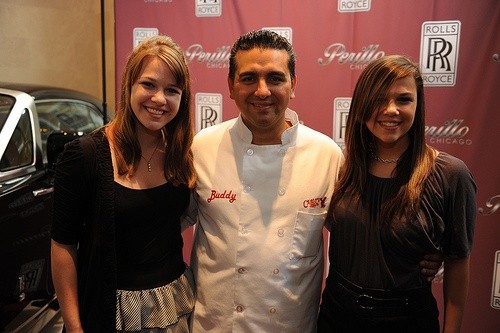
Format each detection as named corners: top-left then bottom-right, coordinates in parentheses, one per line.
top-left (1, 82), bottom-right (113, 332)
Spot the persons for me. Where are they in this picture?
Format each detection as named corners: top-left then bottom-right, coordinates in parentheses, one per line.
top-left (50, 35), bottom-right (197, 333)
top-left (317, 55), bottom-right (477, 333)
top-left (182, 29), bottom-right (443, 333)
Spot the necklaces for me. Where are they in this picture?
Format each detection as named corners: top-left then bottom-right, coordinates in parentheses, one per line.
top-left (371, 151), bottom-right (401, 163)
top-left (141, 137), bottom-right (159, 172)
